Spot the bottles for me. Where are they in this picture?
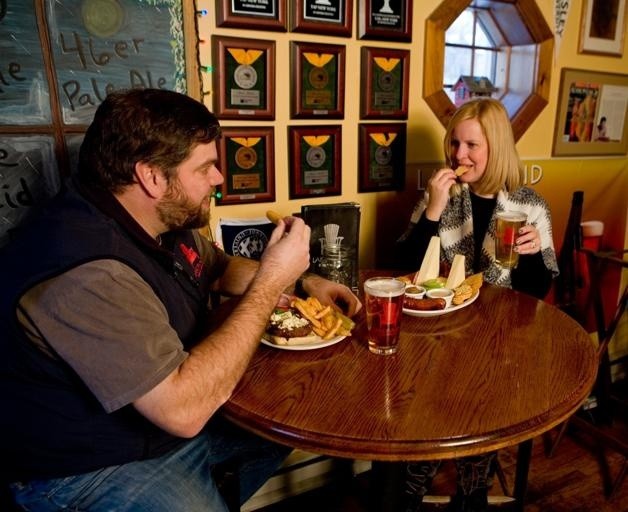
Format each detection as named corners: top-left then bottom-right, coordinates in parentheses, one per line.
top-left (320, 245), bottom-right (353, 291)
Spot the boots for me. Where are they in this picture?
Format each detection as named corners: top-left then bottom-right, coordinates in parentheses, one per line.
top-left (448, 454), bottom-right (495, 512)
top-left (376, 460), bottom-right (441, 512)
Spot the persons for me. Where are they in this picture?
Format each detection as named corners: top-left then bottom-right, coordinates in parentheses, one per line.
top-left (394, 96), bottom-right (562, 512)
top-left (0, 87), bottom-right (364, 512)
top-left (596, 116), bottom-right (608, 140)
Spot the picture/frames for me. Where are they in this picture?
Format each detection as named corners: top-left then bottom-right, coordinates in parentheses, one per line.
top-left (215, 0), bottom-right (288, 33)
top-left (292, 0), bottom-right (353, 38)
top-left (287, 125), bottom-right (342, 200)
top-left (210, 34), bottom-right (276, 121)
top-left (575, 0), bottom-right (626, 58)
top-left (550, 67), bottom-right (628, 156)
top-left (289, 40), bottom-right (346, 120)
top-left (357, 123), bottom-right (407, 193)
top-left (359, 46), bottom-right (411, 120)
top-left (215, 126), bottom-right (276, 207)
top-left (356, 0), bottom-right (413, 43)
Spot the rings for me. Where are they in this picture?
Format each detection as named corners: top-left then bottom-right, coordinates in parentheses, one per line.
top-left (531, 240), bottom-right (536, 248)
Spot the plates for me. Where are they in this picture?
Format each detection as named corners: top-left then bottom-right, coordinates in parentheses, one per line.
top-left (258, 332), bottom-right (352, 352)
top-left (402, 291), bottom-right (481, 320)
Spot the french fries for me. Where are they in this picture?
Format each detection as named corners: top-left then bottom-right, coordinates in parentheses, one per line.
top-left (289, 296), bottom-right (355, 342)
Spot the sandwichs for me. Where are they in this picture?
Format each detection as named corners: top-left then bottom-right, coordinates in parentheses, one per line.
top-left (444, 253), bottom-right (466, 289)
top-left (414, 234), bottom-right (441, 286)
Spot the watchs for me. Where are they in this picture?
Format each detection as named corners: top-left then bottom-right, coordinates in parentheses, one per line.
top-left (294, 272), bottom-right (309, 298)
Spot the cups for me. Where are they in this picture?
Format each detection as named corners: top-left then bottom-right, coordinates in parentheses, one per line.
top-left (494, 212), bottom-right (528, 269)
top-left (363, 277), bottom-right (407, 356)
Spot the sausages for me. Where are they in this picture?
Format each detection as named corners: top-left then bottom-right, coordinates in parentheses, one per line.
top-left (403, 297), bottom-right (447, 311)
